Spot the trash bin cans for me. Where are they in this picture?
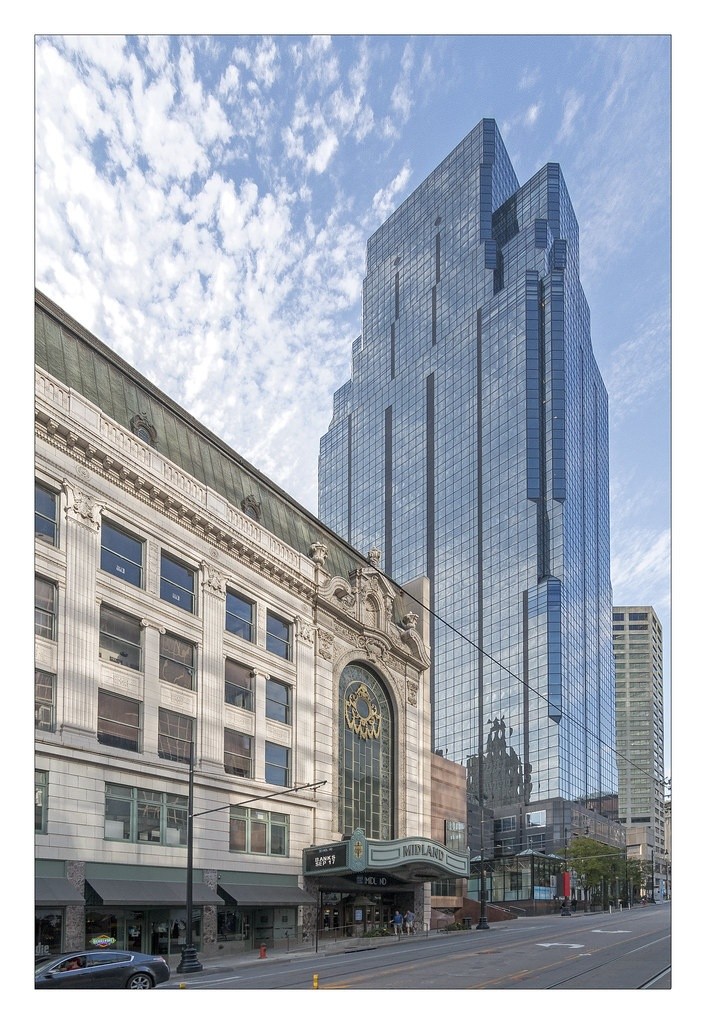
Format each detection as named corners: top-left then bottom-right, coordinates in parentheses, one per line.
top-left (462, 918), bottom-right (471, 929)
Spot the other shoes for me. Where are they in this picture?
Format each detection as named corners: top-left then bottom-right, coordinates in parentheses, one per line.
top-left (395, 933), bottom-right (397, 936)
top-left (412, 932), bottom-right (416, 935)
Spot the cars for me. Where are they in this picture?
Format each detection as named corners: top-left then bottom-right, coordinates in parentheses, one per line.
top-left (34, 949), bottom-right (171, 990)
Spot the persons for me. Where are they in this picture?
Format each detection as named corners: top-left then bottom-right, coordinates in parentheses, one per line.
top-left (570, 897), bottom-right (578, 914)
top-left (59, 957), bottom-right (81, 971)
top-left (388, 911), bottom-right (404, 935)
top-left (404, 910), bottom-right (416, 936)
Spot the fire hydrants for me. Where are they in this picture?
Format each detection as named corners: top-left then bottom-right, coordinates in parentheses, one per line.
top-left (258, 943), bottom-right (267, 960)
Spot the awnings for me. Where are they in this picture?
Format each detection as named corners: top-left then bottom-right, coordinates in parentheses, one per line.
top-left (85, 879), bottom-right (225, 905)
top-left (34, 878), bottom-right (86, 905)
top-left (217, 883), bottom-right (318, 906)
